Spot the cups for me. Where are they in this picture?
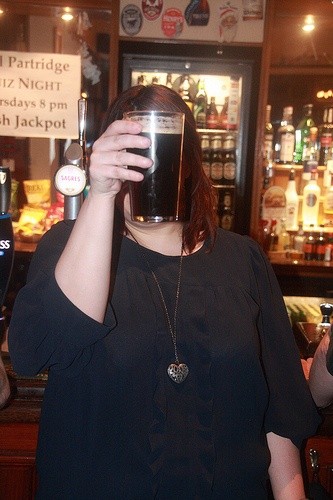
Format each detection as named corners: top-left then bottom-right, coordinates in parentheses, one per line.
top-left (123, 111), bottom-right (185, 222)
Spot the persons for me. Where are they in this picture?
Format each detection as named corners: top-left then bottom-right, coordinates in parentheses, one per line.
top-left (307, 321), bottom-right (333, 410)
top-left (10, 85), bottom-right (322, 499)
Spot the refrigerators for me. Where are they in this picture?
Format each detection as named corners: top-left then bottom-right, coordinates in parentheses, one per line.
top-left (122, 53), bottom-right (255, 235)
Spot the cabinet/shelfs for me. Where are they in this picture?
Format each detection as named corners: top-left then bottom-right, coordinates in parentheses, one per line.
top-left (247, 67), bottom-right (333, 287)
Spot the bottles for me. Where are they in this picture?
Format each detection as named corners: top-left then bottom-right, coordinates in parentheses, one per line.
top-left (264, 218), bottom-right (333, 262)
top-left (260, 165), bottom-right (333, 229)
top-left (200, 133), bottom-right (236, 185)
top-left (137, 73), bottom-right (237, 130)
top-left (264, 104), bottom-right (333, 165)
top-left (222, 192), bottom-right (233, 231)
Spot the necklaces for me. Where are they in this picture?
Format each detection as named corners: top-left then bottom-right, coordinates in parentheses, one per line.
top-left (125, 223), bottom-right (191, 384)
